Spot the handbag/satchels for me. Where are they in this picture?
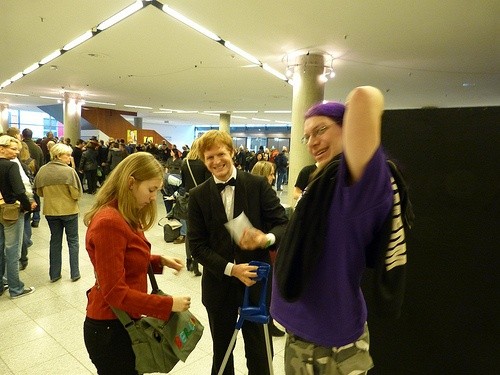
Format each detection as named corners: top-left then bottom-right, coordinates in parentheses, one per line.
top-left (164, 224), bottom-right (181, 244)
top-left (174, 192), bottom-right (190, 220)
top-left (94, 263), bottom-right (205, 374)
top-left (97, 165), bottom-right (103, 177)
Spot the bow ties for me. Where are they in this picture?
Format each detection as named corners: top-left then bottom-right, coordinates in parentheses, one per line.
top-left (216, 177), bottom-right (236, 193)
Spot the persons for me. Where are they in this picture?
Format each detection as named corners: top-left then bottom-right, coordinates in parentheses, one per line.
top-left (19, 142), bottom-right (37, 175)
top-left (251, 161), bottom-right (287, 338)
top-left (269, 84), bottom-right (416, 375)
top-left (22, 129), bottom-right (45, 228)
top-left (293, 161), bottom-right (323, 201)
top-left (34, 143), bottom-right (83, 282)
top-left (83, 153), bottom-right (187, 374)
top-left (36, 131), bottom-right (289, 196)
top-left (186, 129), bottom-right (292, 375)
top-left (1, 135), bottom-right (36, 300)
top-left (2, 133), bottom-right (38, 269)
top-left (9, 126), bottom-right (35, 247)
top-left (182, 139), bottom-right (214, 277)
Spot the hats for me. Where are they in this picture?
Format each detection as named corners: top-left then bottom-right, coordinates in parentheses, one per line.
top-left (89, 135), bottom-right (98, 142)
top-left (0, 135), bottom-right (15, 145)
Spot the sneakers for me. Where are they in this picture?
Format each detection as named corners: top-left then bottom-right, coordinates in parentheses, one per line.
top-left (10, 286), bottom-right (35, 299)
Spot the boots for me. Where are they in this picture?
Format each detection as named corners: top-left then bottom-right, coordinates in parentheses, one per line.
top-left (186, 258), bottom-right (202, 276)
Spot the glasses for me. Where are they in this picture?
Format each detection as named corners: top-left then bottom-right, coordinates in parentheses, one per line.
top-left (301, 120), bottom-right (341, 145)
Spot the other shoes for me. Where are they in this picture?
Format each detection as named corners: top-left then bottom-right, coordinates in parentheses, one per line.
top-left (31, 221), bottom-right (38, 227)
top-left (173, 235), bottom-right (186, 244)
